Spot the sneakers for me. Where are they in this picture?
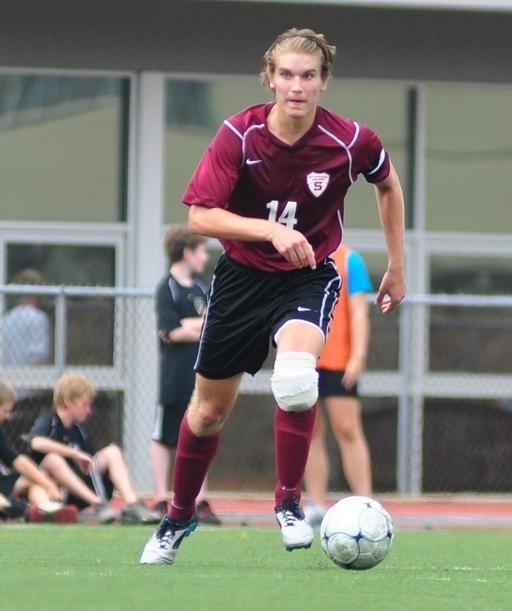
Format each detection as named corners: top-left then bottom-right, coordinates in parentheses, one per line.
top-left (140, 513), bottom-right (199, 566)
top-left (0, 501), bottom-right (79, 523)
top-left (276, 504), bottom-right (315, 551)
top-left (95, 500), bottom-right (219, 528)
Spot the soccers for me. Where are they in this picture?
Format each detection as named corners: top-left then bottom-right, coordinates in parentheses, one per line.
top-left (320, 496), bottom-right (394, 571)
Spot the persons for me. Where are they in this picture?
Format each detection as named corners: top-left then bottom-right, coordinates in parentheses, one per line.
top-left (26, 374), bottom-right (165, 527)
top-left (0, 269), bottom-right (56, 367)
top-left (1, 383), bottom-right (82, 525)
top-left (145, 220), bottom-right (226, 526)
top-left (139, 24), bottom-right (408, 569)
top-left (295, 232), bottom-right (376, 525)
